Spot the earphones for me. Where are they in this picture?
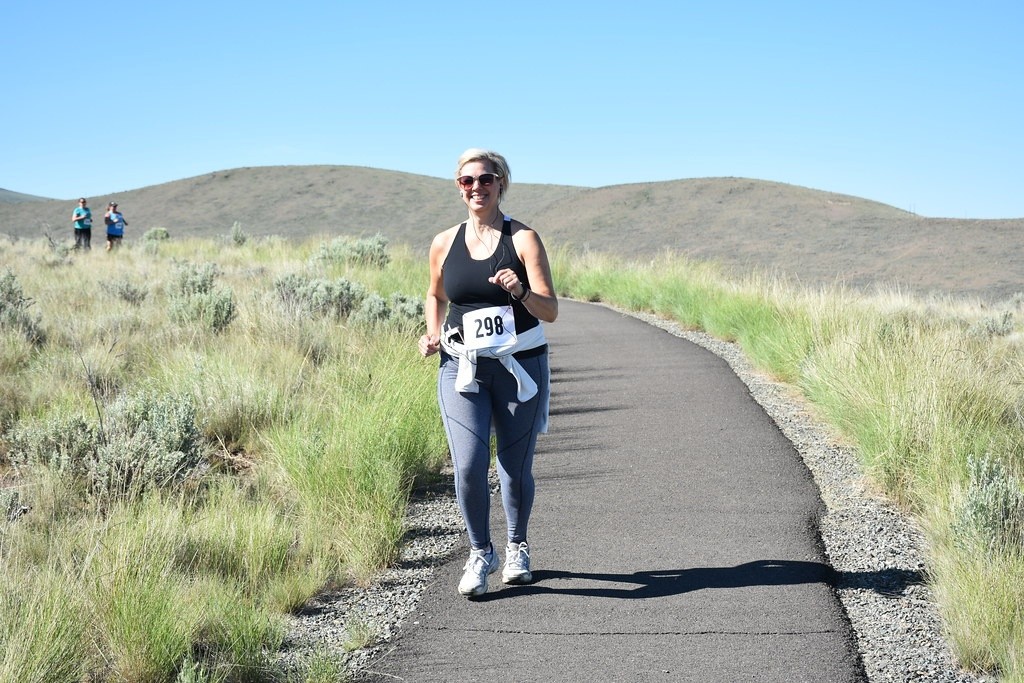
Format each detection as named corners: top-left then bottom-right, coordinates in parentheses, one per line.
top-left (499, 184), bottom-right (503, 194)
top-left (460, 191), bottom-right (463, 197)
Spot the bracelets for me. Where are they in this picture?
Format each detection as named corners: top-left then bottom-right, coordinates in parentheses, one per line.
top-left (522, 289), bottom-right (530, 303)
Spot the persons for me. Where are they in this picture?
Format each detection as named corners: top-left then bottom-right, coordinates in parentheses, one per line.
top-left (72, 198), bottom-right (93, 249)
top-left (417, 148), bottom-right (558, 596)
top-left (104, 201), bottom-right (128, 252)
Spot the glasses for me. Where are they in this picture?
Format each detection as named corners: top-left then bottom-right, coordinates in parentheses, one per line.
top-left (456, 173), bottom-right (504, 190)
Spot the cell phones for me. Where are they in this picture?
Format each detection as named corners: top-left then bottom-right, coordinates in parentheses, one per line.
top-left (445, 326), bottom-right (466, 346)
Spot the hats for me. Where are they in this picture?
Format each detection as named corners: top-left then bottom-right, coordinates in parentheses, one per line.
top-left (78, 198), bottom-right (86, 203)
top-left (109, 201), bottom-right (118, 207)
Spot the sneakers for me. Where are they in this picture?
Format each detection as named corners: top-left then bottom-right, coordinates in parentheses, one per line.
top-left (501, 542), bottom-right (532, 585)
top-left (458, 541), bottom-right (499, 597)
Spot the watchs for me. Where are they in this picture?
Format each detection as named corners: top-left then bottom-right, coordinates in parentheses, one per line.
top-left (511, 281), bottom-right (527, 301)
